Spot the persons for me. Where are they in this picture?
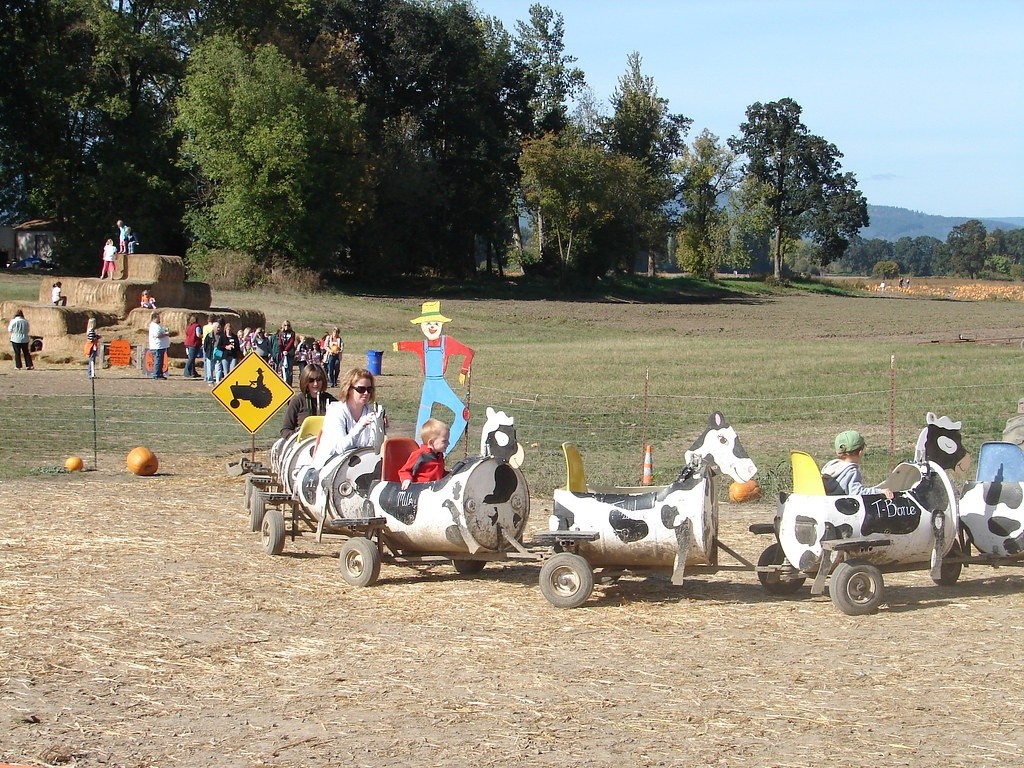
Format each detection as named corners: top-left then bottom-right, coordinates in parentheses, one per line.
top-left (881, 280), bottom-right (886, 291)
top-left (8, 310), bottom-right (34, 370)
top-left (117, 220), bottom-right (140, 254)
top-left (184, 315), bottom-right (344, 388)
top-left (86, 318), bottom-right (101, 379)
top-left (899, 277), bottom-right (904, 288)
top-left (52, 282), bottom-right (67, 306)
top-left (398, 419), bottom-right (451, 491)
top-left (149, 313), bottom-right (170, 380)
top-left (311, 368), bottom-right (388, 471)
top-left (141, 290), bottom-right (157, 309)
top-left (819, 430), bottom-right (894, 500)
top-left (906, 278), bottom-right (910, 288)
top-left (280, 364), bottom-right (338, 441)
top-left (100, 239), bottom-right (117, 280)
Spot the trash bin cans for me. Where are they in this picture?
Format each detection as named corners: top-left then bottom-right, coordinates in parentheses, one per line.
top-left (368, 350), bottom-right (384, 376)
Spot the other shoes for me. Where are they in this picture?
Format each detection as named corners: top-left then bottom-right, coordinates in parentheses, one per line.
top-left (26, 366), bottom-right (34, 370)
top-left (14, 367), bottom-right (20, 370)
top-left (154, 375), bottom-right (167, 380)
top-left (207, 380), bottom-right (214, 383)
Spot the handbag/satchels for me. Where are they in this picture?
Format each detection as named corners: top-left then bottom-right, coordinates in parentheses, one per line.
top-left (280, 355), bottom-right (288, 369)
top-left (213, 347), bottom-right (223, 360)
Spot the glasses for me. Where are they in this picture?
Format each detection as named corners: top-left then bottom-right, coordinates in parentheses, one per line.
top-left (308, 375), bottom-right (322, 383)
top-left (349, 386), bottom-right (374, 393)
top-left (284, 325), bottom-right (289, 326)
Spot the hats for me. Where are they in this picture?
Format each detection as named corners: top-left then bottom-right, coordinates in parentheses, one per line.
top-left (1017, 398), bottom-right (1024, 413)
top-left (835, 430), bottom-right (865, 453)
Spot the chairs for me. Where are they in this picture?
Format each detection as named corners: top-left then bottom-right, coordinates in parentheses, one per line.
top-left (296, 415), bottom-right (325, 442)
top-left (562, 441), bottom-right (590, 493)
top-left (976, 440), bottom-right (1024, 482)
top-left (382, 437), bottom-right (420, 481)
top-left (790, 450), bottom-right (826, 494)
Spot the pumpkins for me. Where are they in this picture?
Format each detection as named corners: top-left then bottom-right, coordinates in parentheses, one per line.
top-left (331, 344), bottom-right (339, 353)
top-left (127, 447), bottom-right (158, 476)
top-left (65, 456), bottom-right (83, 472)
top-left (729, 479), bottom-right (761, 503)
